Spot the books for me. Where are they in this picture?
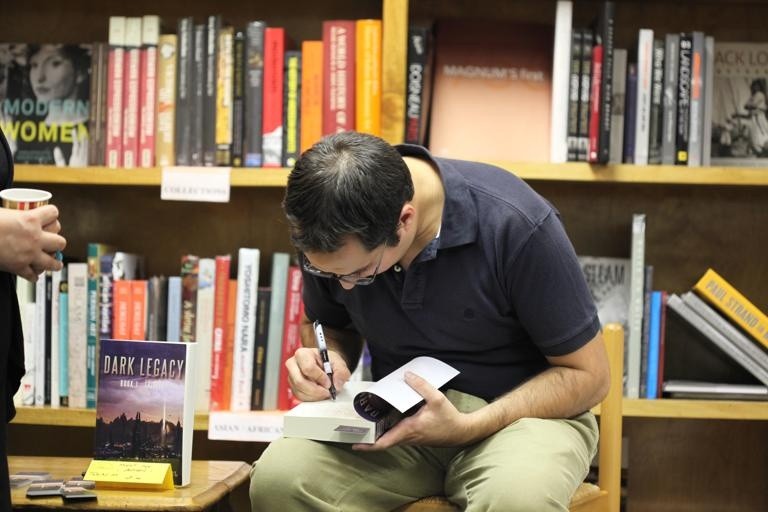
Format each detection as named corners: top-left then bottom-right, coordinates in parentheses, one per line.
top-left (283, 355), bottom-right (462, 444)
top-left (1, 0), bottom-right (434, 168)
top-left (578, 215), bottom-right (768, 399)
top-left (551, 1), bottom-right (768, 166)
top-left (12, 242), bottom-right (372, 413)
top-left (8, 471), bottom-right (97, 500)
top-left (95, 339), bottom-right (199, 488)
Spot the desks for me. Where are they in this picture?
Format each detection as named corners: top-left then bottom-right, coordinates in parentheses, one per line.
top-left (4, 453), bottom-right (255, 510)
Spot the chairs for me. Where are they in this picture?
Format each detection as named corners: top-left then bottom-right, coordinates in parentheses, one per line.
top-left (407, 325), bottom-right (626, 511)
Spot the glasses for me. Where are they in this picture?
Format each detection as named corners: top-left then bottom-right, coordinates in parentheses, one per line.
top-left (303, 236), bottom-right (389, 287)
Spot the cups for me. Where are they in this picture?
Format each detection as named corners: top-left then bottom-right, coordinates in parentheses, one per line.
top-left (111, 250), bottom-right (137, 282)
top-left (1, 185), bottom-right (52, 209)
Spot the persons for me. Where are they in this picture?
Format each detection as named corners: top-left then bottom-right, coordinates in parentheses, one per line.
top-left (0, 131), bottom-right (66, 512)
top-left (249, 132), bottom-right (611, 512)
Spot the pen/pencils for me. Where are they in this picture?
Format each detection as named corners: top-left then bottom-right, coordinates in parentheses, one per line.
top-left (313, 319), bottom-right (336, 400)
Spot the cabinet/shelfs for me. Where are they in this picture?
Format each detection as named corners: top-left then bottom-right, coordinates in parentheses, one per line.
top-left (0, 2), bottom-right (768, 436)
